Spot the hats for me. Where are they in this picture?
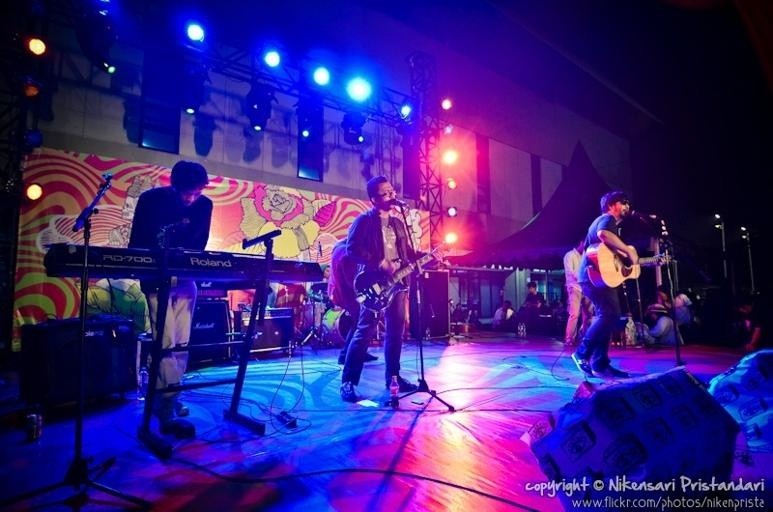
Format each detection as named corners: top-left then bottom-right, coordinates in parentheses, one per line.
top-left (646, 303), bottom-right (667, 313)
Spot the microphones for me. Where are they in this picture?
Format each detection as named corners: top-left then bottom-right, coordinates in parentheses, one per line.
top-left (319, 244), bottom-right (322, 258)
top-left (390, 197), bottom-right (408, 212)
top-left (100, 168), bottom-right (113, 186)
top-left (627, 208), bottom-right (649, 225)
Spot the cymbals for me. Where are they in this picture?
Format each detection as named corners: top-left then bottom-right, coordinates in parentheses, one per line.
top-left (321, 307), bottom-right (351, 347)
top-left (310, 282), bottom-right (329, 291)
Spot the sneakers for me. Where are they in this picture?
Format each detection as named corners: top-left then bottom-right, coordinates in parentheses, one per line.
top-left (385, 376), bottom-right (417, 393)
top-left (592, 365), bottom-right (629, 381)
top-left (340, 381), bottom-right (355, 402)
top-left (571, 352), bottom-right (592, 376)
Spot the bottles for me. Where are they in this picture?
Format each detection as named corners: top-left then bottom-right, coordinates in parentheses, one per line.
top-left (136, 367), bottom-right (149, 401)
top-left (516, 322), bottom-right (526, 337)
top-left (425, 327), bottom-right (431, 341)
top-left (389, 375), bottom-right (399, 409)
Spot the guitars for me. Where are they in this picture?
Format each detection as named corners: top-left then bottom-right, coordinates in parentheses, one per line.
top-left (586, 242), bottom-right (672, 289)
top-left (353, 240), bottom-right (452, 312)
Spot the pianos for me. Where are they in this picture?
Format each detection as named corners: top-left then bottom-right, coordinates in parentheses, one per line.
top-left (43, 241), bottom-right (326, 297)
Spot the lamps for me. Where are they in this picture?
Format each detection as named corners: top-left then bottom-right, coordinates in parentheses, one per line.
top-left (175, 64), bottom-right (369, 147)
top-left (99, 60), bottom-right (118, 76)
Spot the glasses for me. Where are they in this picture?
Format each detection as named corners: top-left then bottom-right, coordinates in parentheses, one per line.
top-left (620, 199), bottom-right (632, 206)
top-left (380, 190), bottom-right (397, 197)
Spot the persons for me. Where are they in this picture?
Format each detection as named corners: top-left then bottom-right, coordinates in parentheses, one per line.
top-left (308, 240), bottom-right (330, 366)
top-left (337, 175), bottom-right (445, 405)
top-left (569, 189), bottom-right (671, 379)
top-left (563, 237), bottom-right (594, 344)
top-left (447, 283), bottom-right (763, 351)
top-left (326, 237), bottom-right (380, 365)
top-left (128, 161), bottom-right (213, 419)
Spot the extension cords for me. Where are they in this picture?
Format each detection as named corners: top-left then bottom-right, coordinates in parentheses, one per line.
top-left (276, 411), bottom-right (296, 426)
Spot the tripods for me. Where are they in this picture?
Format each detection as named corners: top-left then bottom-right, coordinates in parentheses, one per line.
top-left (7, 187), bottom-right (155, 512)
top-left (300, 246), bottom-right (325, 350)
top-left (381, 207), bottom-right (457, 412)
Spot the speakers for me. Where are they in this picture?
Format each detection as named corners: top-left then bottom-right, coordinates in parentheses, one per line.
top-left (419, 269), bottom-right (451, 340)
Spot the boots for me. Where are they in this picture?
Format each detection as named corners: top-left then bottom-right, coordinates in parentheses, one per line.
top-left (158, 390), bottom-right (195, 436)
top-left (154, 394), bottom-right (188, 416)
